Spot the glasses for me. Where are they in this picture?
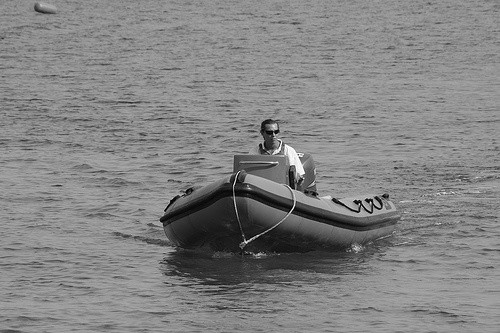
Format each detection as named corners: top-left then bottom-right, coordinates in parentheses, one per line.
top-left (266, 130), bottom-right (279, 134)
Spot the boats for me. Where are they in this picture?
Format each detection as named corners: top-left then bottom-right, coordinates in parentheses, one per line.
top-left (159, 153), bottom-right (399, 251)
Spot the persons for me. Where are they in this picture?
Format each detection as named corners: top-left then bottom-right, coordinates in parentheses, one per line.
top-left (249, 119), bottom-right (306, 186)
